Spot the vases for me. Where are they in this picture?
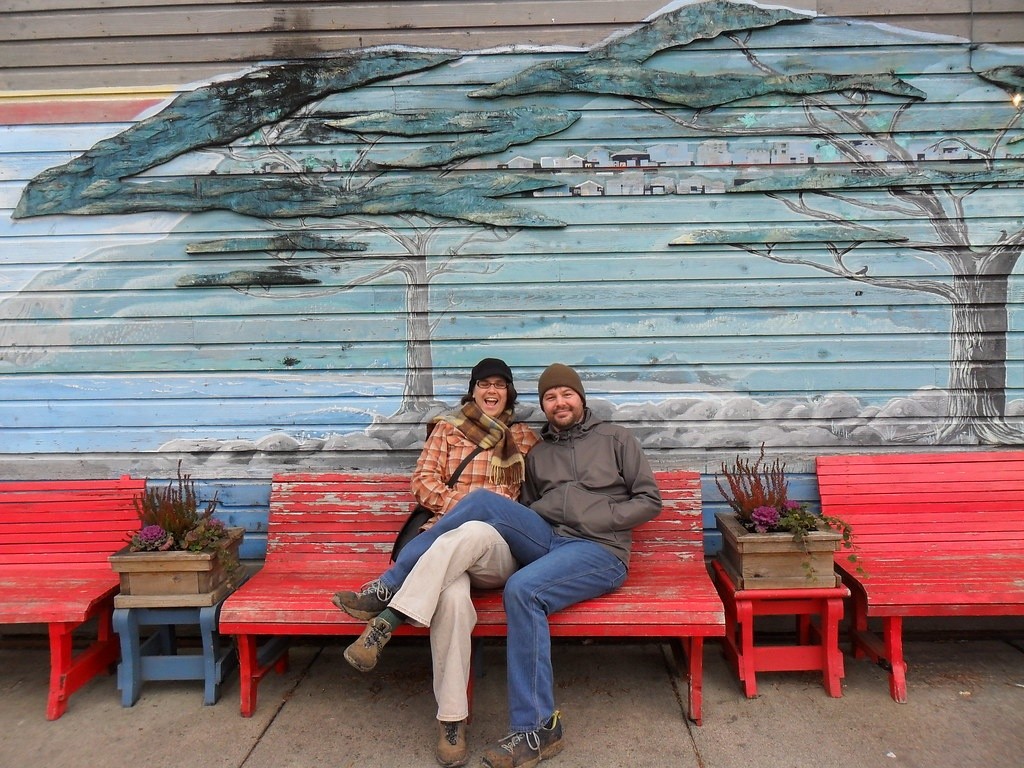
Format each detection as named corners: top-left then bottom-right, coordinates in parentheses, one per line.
top-left (715, 513), bottom-right (843, 590)
top-left (108, 528), bottom-right (246, 608)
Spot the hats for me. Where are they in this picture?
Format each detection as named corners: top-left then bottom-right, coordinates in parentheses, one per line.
top-left (537, 362), bottom-right (586, 412)
top-left (471, 358), bottom-right (513, 382)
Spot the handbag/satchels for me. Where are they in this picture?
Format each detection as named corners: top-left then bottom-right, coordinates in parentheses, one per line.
top-left (388, 502), bottom-right (436, 566)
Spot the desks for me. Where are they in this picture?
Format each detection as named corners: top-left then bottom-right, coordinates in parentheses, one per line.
top-left (711, 560), bottom-right (852, 699)
top-left (112, 574), bottom-right (249, 705)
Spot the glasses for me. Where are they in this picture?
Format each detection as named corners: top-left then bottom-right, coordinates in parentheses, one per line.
top-left (475, 379), bottom-right (507, 389)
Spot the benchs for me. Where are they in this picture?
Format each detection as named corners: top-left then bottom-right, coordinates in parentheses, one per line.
top-left (1, 480), bottom-right (146, 723)
top-left (815, 452), bottom-right (1024, 705)
top-left (219, 470), bottom-right (726, 721)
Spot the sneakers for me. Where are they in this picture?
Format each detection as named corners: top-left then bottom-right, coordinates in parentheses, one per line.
top-left (343, 616), bottom-right (394, 673)
top-left (330, 576), bottom-right (395, 621)
top-left (482, 710), bottom-right (563, 767)
top-left (435, 717), bottom-right (469, 768)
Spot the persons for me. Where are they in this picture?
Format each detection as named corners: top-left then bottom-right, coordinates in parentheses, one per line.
top-left (344, 357), bottom-right (543, 768)
top-left (332, 362), bottom-right (662, 768)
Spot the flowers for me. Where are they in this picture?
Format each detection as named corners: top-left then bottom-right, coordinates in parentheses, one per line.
top-left (108, 457), bottom-right (243, 590)
top-left (715, 441), bottom-right (870, 584)
